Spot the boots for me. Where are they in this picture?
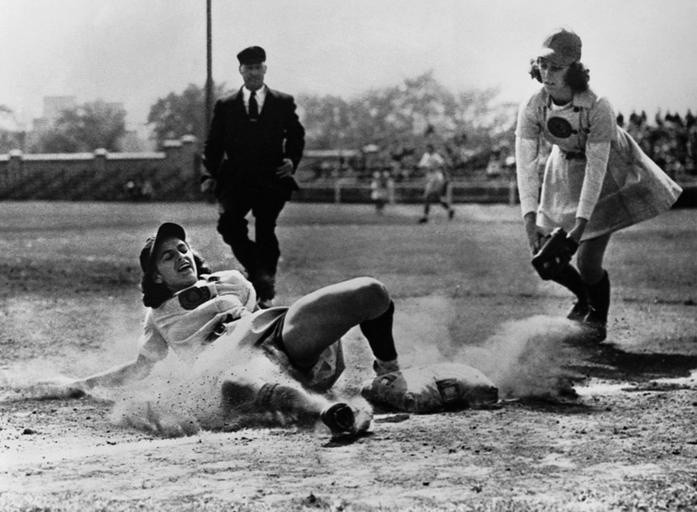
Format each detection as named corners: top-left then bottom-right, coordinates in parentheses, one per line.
top-left (551, 263), bottom-right (590, 321)
top-left (563, 267), bottom-right (610, 345)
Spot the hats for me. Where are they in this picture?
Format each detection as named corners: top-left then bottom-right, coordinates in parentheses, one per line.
top-left (535, 27), bottom-right (583, 67)
top-left (236, 45), bottom-right (267, 66)
top-left (138, 221), bottom-right (187, 274)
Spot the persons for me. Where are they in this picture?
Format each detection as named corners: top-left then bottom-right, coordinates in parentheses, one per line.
top-left (370, 171), bottom-right (394, 208)
top-left (61, 221), bottom-right (401, 441)
top-left (413, 143), bottom-right (454, 226)
top-left (199, 46), bottom-right (307, 310)
top-left (513, 28), bottom-right (684, 348)
top-left (368, 107), bottom-right (697, 186)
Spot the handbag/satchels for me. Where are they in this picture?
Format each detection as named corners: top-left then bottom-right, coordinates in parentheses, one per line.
top-left (351, 361), bottom-right (500, 413)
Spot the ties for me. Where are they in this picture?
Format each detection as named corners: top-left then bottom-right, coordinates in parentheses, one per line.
top-left (248, 90), bottom-right (259, 120)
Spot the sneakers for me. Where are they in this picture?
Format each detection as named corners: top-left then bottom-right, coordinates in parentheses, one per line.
top-left (319, 402), bottom-right (355, 435)
